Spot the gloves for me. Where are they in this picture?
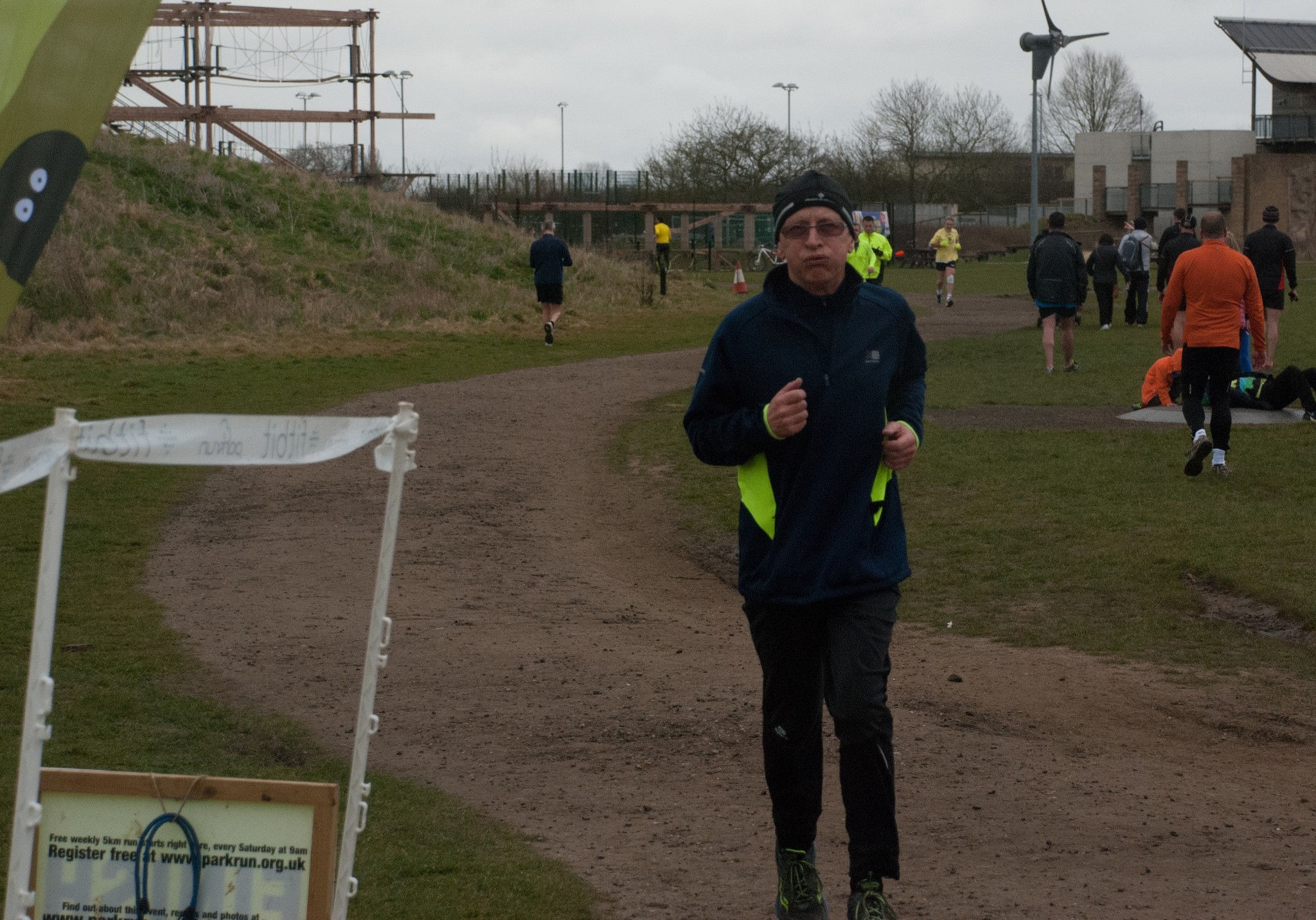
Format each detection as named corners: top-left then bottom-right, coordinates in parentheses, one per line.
top-left (871, 248), bottom-right (883, 257)
top-left (955, 243), bottom-right (961, 250)
top-left (940, 241), bottom-right (949, 247)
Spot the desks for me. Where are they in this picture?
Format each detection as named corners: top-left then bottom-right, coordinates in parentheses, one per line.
top-left (900, 248), bottom-right (934, 269)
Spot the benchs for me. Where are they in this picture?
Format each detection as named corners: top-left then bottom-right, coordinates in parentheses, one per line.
top-left (901, 256), bottom-right (937, 262)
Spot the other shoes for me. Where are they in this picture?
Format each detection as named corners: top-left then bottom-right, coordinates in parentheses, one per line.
top-left (1132, 403), bottom-right (1141, 410)
top-left (937, 295), bottom-right (941, 303)
top-left (1062, 360), bottom-right (1079, 372)
top-left (1137, 324), bottom-right (1146, 327)
top-left (1100, 323), bottom-right (1111, 330)
top-left (544, 322), bottom-right (553, 344)
top-left (946, 300), bottom-right (954, 307)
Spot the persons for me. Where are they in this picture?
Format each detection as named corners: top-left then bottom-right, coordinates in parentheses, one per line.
top-left (1025, 205), bottom-right (1316, 478)
top-left (528, 221), bottom-right (572, 347)
top-left (680, 175), bottom-right (932, 920)
top-left (654, 217), bottom-right (672, 274)
top-left (846, 215), bottom-right (893, 287)
top-left (929, 218), bottom-right (961, 307)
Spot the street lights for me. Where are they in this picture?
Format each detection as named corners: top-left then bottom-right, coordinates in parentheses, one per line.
top-left (772, 82), bottom-right (799, 173)
top-left (384, 70), bottom-right (414, 181)
top-left (557, 100), bottom-right (569, 170)
top-left (295, 91), bottom-right (322, 166)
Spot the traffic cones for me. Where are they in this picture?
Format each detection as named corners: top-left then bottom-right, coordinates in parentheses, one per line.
top-left (732, 259), bottom-right (749, 295)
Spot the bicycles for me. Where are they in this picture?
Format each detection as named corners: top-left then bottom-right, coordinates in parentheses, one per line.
top-left (747, 242), bottom-right (788, 273)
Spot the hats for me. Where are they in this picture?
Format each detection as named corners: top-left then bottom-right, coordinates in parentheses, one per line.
top-left (772, 170), bottom-right (856, 242)
top-left (1181, 215), bottom-right (1197, 229)
top-left (1262, 206), bottom-right (1279, 223)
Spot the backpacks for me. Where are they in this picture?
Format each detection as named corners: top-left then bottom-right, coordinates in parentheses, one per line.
top-left (1121, 235), bottom-right (1149, 272)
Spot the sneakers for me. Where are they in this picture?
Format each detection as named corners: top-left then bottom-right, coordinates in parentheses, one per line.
top-left (1304, 410), bottom-right (1316, 422)
top-left (1184, 435), bottom-right (1212, 477)
top-left (1212, 464), bottom-right (1231, 476)
top-left (847, 871), bottom-right (897, 920)
top-left (775, 848), bottom-right (829, 920)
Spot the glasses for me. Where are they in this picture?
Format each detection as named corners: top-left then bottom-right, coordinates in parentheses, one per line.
top-left (780, 223), bottom-right (849, 239)
